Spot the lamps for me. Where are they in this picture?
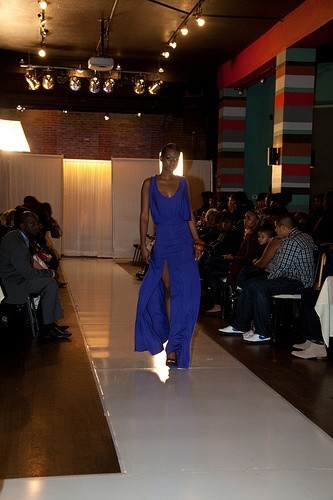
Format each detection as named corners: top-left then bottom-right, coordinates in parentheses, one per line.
top-left (25, 65), bottom-right (40, 90)
top-left (103, 79), bottom-right (115, 93)
top-left (260, 77), bottom-right (267, 84)
top-left (148, 81), bottom-right (166, 95)
top-left (134, 72), bottom-right (146, 94)
top-left (69, 69), bottom-right (81, 91)
top-left (42, 67), bottom-right (54, 89)
top-left (89, 69), bottom-right (101, 93)
top-left (237, 87), bottom-right (244, 96)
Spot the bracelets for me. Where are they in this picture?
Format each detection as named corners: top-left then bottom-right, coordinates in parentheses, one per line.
top-left (194, 239), bottom-right (202, 245)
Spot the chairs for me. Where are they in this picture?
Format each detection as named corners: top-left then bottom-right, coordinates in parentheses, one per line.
top-left (272, 253), bottom-right (327, 343)
top-left (132, 234), bottom-right (153, 265)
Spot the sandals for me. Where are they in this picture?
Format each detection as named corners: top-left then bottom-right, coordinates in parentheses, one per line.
top-left (166, 359), bottom-right (175, 367)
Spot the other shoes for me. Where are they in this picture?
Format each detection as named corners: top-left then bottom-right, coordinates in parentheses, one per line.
top-left (218, 325), bottom-right (245, 334)
top-left (58, 282), bottom-right (67, 288)
top-left (243, 329), bottom-right (271, 342)
top-left (291, 342), bottom-right (328, 360)
top-left (136, 271), bottom-right (145, 280)
top-left (205, 304), bottom-right (221, 313)
top-left (292, 340), bottom-right (312, 350)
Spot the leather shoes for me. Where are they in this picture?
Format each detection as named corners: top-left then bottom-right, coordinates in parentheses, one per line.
top-left (42, 322), bottom-right (72, 338)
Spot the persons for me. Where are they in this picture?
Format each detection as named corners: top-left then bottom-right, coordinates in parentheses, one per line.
top-left (242, 216), bottom-right (315, 341)
top-left (134, 144), bottom-right (206, 370)
top-left (0, 211), bottom-right (72, 339)
top-left (0, 196), bottom-right (67, 289)
top-left (135, 190), bottom-right (292, 316)
top-left (293, 289), bottom-right (327, 359)
